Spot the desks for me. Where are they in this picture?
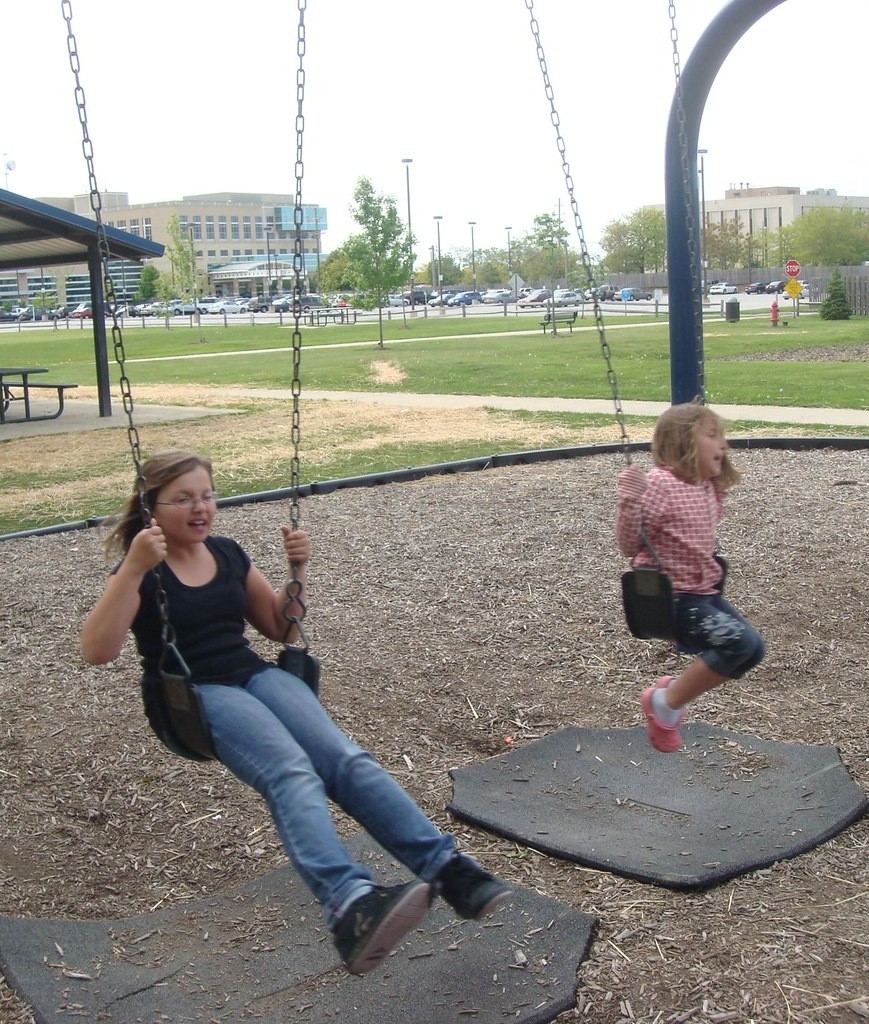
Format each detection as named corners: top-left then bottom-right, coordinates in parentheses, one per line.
top-left (0, 367), bottom-right (49, 421)
top-left (305, 307), bottom-right (355, 327)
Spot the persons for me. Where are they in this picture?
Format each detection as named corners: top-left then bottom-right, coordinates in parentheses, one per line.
top-left (615, 403), bottom-right (765, 752)
top-left (81, 452), bottom-right (512, 974)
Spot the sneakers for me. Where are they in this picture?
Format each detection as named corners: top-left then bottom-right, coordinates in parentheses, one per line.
top-left (439, 857), bottom-right (514, 921)
top-left (332, 880), bottom-right (433, 976)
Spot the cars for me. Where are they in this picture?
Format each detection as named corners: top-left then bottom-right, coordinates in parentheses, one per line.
top-left (482, 292), bottom-right (518, 304)
top-left (427, 293), bottom-right (458, 307)
top-left (518, 289), bottom-right (552, 308)
top-left (447, 292), bottom-right (484, 308)
top-left (782, 280), bottom-right (811, 299)
top-left (543, 291), bottom-right (586, 308)
top-left (479, 288), bottom-right (535, 299)
top-left (700, 280), bottom-right (719, 293)
top-left (744, 282), bottom-right (767, 295)
top-left (765, 280), bottom-right (789, 292)
top-left (0, 290), bottom-right (378, 323)
top-left (709, 282), bottom-right (739, 293)
top-left (380, 294), bottom-right (411, 308)
top-left (557, 284), bottom-right (654, 302)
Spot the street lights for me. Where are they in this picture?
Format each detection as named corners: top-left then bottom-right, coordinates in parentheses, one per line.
top-left (433, 216), bottom-right (456, 316)
top-left (468, 221), bottom-right (479, 306)
top-left (313, 207), bottom-right (324, 295)
top-left (401, 158), bottom-right (418, 310)
top-left (505, 226), bottom-right (515, 291)
top-left (263, 225), bottom-right (280, 314)
top-left (697, 148), bottom-right (714, 306)
top-left (187, 221), bottom-right (202, 323)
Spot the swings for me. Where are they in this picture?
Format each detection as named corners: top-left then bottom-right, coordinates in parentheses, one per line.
top-left (57, 0), bottom-right (324, 764)
top-left (521, 0), bottom-right (731, 644)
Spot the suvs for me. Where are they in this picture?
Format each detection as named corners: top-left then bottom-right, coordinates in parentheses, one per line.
top-left (402, 289), bottom-right (438, 306)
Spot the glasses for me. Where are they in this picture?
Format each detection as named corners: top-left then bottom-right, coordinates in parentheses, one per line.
top-left (151, 490), bottom-right (219, 511)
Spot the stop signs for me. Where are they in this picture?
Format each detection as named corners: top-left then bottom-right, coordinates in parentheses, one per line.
top-left (784, 260), bottom-right (800, 278)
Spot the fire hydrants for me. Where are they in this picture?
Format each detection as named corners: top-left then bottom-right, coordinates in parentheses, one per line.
top-left (770, 301), bottom-right (782, 328)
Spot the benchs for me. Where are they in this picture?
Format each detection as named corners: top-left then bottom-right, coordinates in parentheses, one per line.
top-left (294, 312), bottom-right (362, 317)
top-left (2, 380), bottom-right (79, 389)
top-left (538, 311), bottom-right (576, 334)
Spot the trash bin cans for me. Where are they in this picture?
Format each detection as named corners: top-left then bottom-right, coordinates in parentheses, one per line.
top-left (724, 302), bottom-right (741, 322)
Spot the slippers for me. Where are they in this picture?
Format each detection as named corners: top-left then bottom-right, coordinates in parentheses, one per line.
top-left (642, 676), bottom-right (687, 751)
